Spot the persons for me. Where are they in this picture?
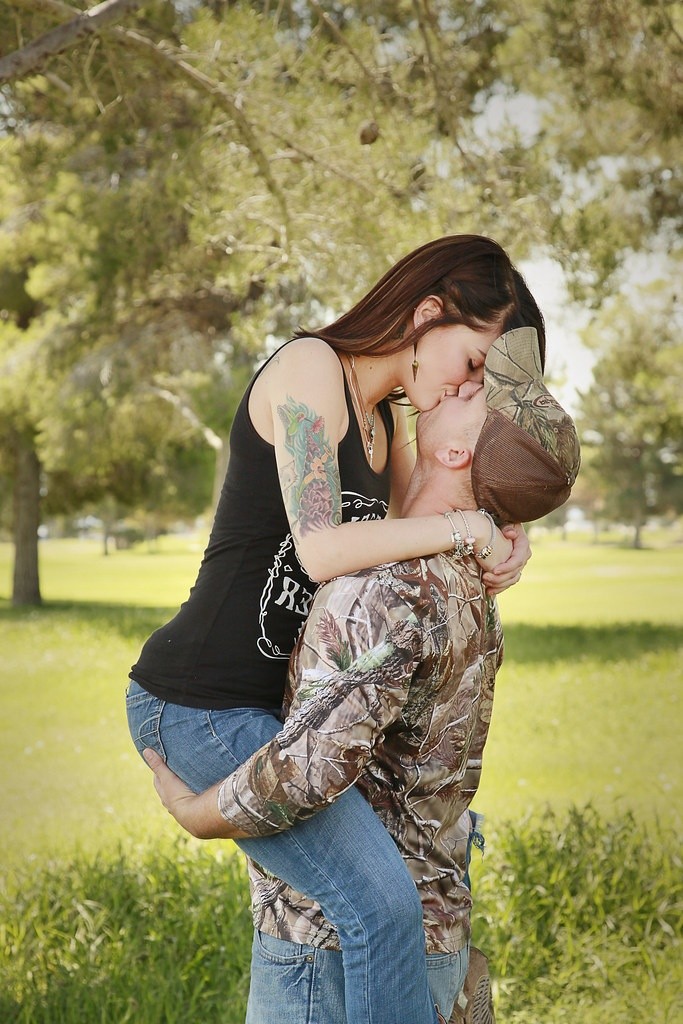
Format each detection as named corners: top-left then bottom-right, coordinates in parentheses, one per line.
top-left (142, 328), bottom-right (582, 1024)
top-left (125, 234), bottom-right (548, 1024)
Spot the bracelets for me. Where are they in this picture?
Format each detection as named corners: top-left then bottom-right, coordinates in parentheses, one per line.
top-left (444, 508), bottom-right (495, 560)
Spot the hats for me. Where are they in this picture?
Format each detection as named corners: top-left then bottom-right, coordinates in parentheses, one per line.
top-left (471, 326), bottom-right (581, 524)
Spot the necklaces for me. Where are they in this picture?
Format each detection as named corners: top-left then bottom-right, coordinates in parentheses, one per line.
top-left (347, 354), bottom-right (376, 467)
